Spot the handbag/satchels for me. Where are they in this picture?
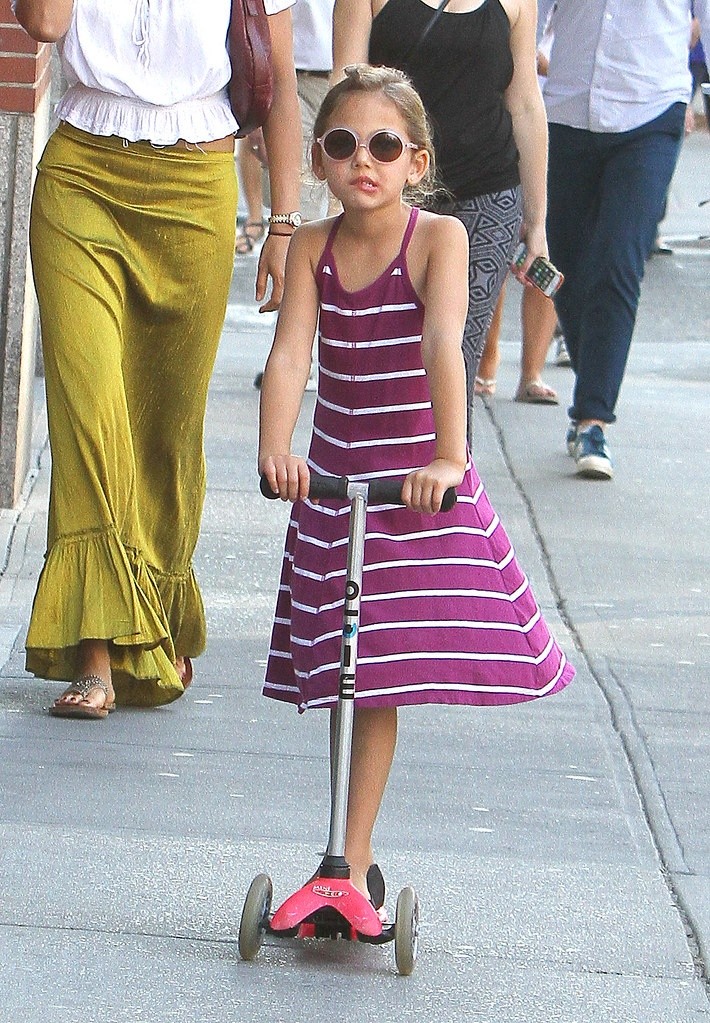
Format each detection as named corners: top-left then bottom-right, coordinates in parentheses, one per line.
top-left (226, 0), bottom-right (274, 138)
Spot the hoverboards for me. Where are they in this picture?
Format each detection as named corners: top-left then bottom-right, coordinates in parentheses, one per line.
top-left (238, 473), bottom-right (456, 975)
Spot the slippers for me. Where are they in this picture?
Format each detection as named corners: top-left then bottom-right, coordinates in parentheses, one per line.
top-left (48, 675), bottom-right (116, 718)
top-left (515, 381), bottom-right (559, 404)
top-left (473, 376), bottom-right (497, 395)
top-left (172, 654), bottom-right (193, 689)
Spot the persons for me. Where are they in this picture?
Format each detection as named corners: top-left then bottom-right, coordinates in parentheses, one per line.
top-left (237, 0), bottom-right (335, 392)
top-left (10, 0), bottom-right (300, 718)
top-left (475, 0), bottom-right (710, 482)
top-left (258, 63), bottom-right (577, 922)
top-left (331, 0), bottom-right (550, 456)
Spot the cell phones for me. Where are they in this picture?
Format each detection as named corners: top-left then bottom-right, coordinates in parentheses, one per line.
top-left (513, 243), bottom-right (564, 297)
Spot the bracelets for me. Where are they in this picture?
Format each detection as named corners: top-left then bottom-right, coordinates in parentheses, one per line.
top-left (268, 231), bottom-right (293, 236)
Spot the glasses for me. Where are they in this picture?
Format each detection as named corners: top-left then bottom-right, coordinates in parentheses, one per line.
top-left (317, 128), bottom-right (418, 164)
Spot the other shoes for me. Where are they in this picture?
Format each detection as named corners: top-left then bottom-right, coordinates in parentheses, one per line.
top-left (566, 420), bottom-right (613, 480)
top-left (653, 241), bottom-right (672, 254)
top-left (556, 339), bottom-right (572, 367)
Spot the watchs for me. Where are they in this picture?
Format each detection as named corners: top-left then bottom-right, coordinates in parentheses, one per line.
top-left (268, 211), bottom-right (302, 229)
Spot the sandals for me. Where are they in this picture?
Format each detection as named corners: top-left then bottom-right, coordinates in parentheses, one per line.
top-left (234, 216), bottom-right (264, 254)
